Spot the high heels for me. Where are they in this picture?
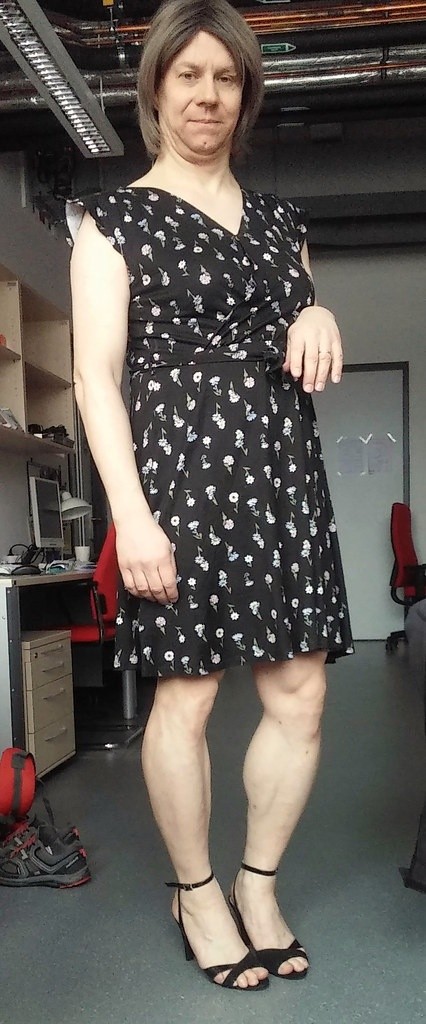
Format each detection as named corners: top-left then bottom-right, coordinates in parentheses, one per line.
top-left (163, 861), bottom-right (311, 993)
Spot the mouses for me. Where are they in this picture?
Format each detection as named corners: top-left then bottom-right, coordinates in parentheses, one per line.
top-left (11, 566), bottom-right (42, 575)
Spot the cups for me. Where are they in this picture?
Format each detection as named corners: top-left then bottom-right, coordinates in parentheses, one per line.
top-left (74, 546), bottom-right (90, 563)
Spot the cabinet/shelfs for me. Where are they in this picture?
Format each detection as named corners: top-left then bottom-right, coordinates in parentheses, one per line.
top-left (1, 278), bottom-right (108, 573)
top-left (20, 630), bottom-right (76, 779)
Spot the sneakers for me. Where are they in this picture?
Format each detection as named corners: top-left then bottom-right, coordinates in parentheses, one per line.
top-left (0, 821), bottom-right (94, 888)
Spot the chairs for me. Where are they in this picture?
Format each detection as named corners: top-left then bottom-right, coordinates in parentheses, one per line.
top-left (34, 519), bottom-right (146, 749)
top-left (385, 501), bottom-right (426, 651)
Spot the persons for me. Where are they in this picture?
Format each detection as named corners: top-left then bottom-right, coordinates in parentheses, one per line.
top-left (66, 0), bottom-right (355, 991)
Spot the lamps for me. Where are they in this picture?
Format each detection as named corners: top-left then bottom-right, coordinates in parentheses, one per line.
top-left (0, 0), bottom-right (124, 158)
top-left (60, 491), bottom-right (93, 521)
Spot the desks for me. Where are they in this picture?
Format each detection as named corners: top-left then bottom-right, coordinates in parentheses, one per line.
top-left (0, 569), bottom-right (99, 753)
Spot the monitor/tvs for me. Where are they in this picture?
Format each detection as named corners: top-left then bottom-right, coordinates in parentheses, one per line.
top-left (22, 477), bottom-right (65, 567)
